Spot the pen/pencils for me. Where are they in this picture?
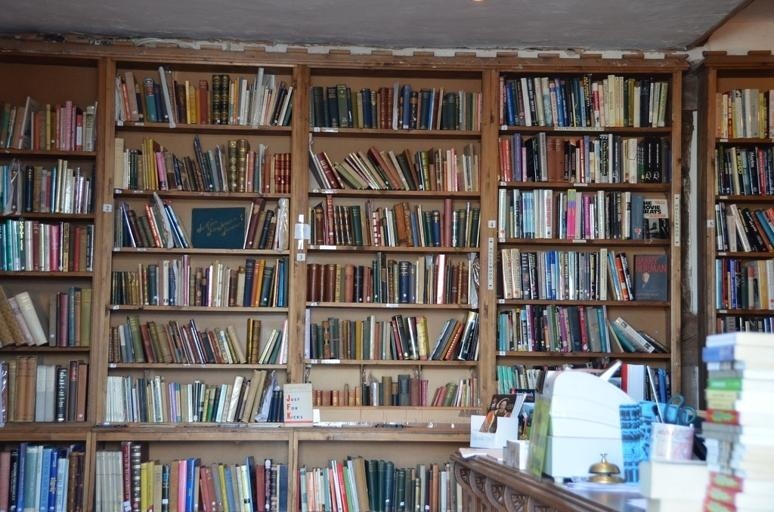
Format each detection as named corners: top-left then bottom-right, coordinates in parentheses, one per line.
top-left (647, 366), bottom-right (667, 423)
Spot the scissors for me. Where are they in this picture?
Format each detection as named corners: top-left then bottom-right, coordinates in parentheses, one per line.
top-left (664, 395), bottom-right (697, 426)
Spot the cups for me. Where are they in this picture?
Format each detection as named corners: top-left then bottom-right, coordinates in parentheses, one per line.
top-left (650, 422), bottom-right (694, 462)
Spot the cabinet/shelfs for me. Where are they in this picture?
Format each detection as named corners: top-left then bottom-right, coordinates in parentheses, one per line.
top-left (0, 38), bottom-right (108, 429)
top-left (491, 59), bottom-right (692, 431)
top-left (302, 50), bottom-right (492, 430)
top-left (108, 43), bottom-right (302, 429)
top-left (699, 59), bottom-right (773, 416)
top-left (1, 429), bottom-right (475, 512)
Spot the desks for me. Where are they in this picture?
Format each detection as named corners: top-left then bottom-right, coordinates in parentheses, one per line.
top-left (450, 451), bottom-right (649, 511)
